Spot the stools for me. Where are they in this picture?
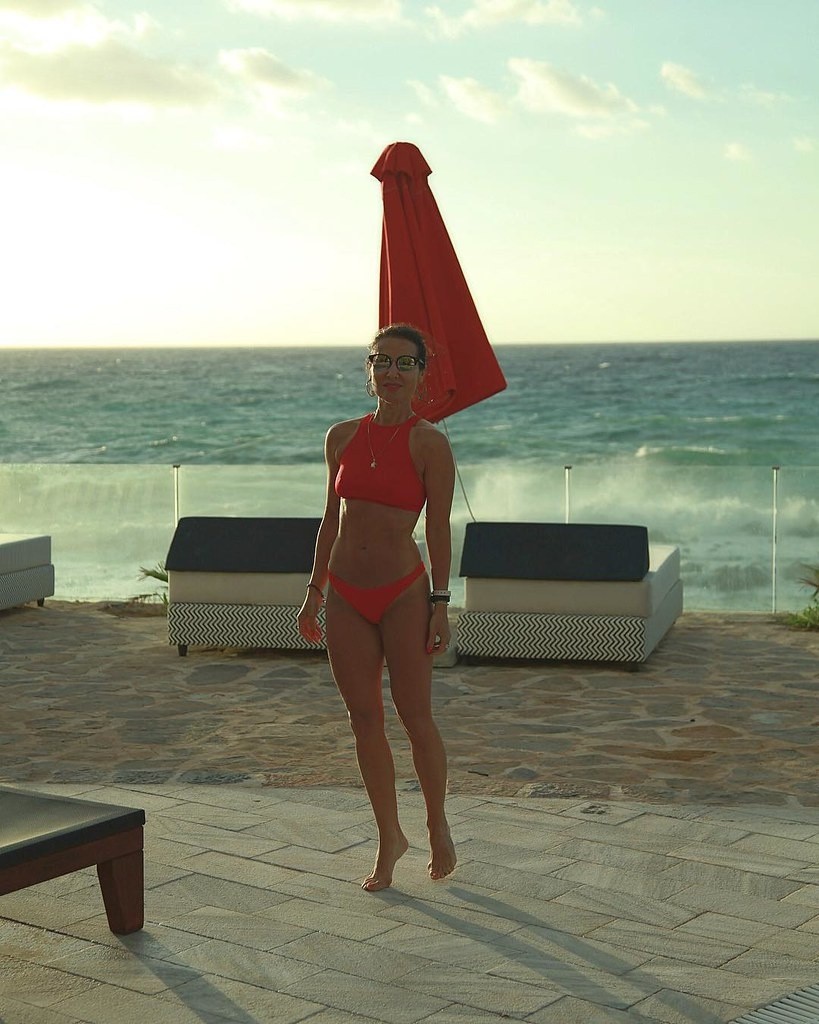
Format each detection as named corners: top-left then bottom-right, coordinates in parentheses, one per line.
top-left (0, 785), bottom-right (146, 939)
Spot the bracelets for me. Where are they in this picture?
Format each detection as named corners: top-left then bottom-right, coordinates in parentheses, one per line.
top-left (430, 590), bottom-right (451, 605)
top-left (306, 584), bottom-right (324, 599)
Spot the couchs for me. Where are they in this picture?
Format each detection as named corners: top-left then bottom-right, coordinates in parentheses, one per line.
top-left (0, 532), bottom-right (58, 616)
top-left (455, 518), bottom-right (685, 673)
top-left (166, 517), bottom-right (350, 656)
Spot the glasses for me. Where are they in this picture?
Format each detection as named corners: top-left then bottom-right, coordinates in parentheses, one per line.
top-left (369, 353), bottom-right (423, 372)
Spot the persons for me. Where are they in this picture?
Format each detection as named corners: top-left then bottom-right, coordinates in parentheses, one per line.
top-left (295, 326), bottom-right (457, 890)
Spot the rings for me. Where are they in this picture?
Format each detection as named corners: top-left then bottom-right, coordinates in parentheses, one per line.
top-left (446, 643), bottom-right (449, 648)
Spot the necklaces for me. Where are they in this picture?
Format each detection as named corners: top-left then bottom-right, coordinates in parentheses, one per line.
top-left (368, 409), bottom-right (414, 469)
